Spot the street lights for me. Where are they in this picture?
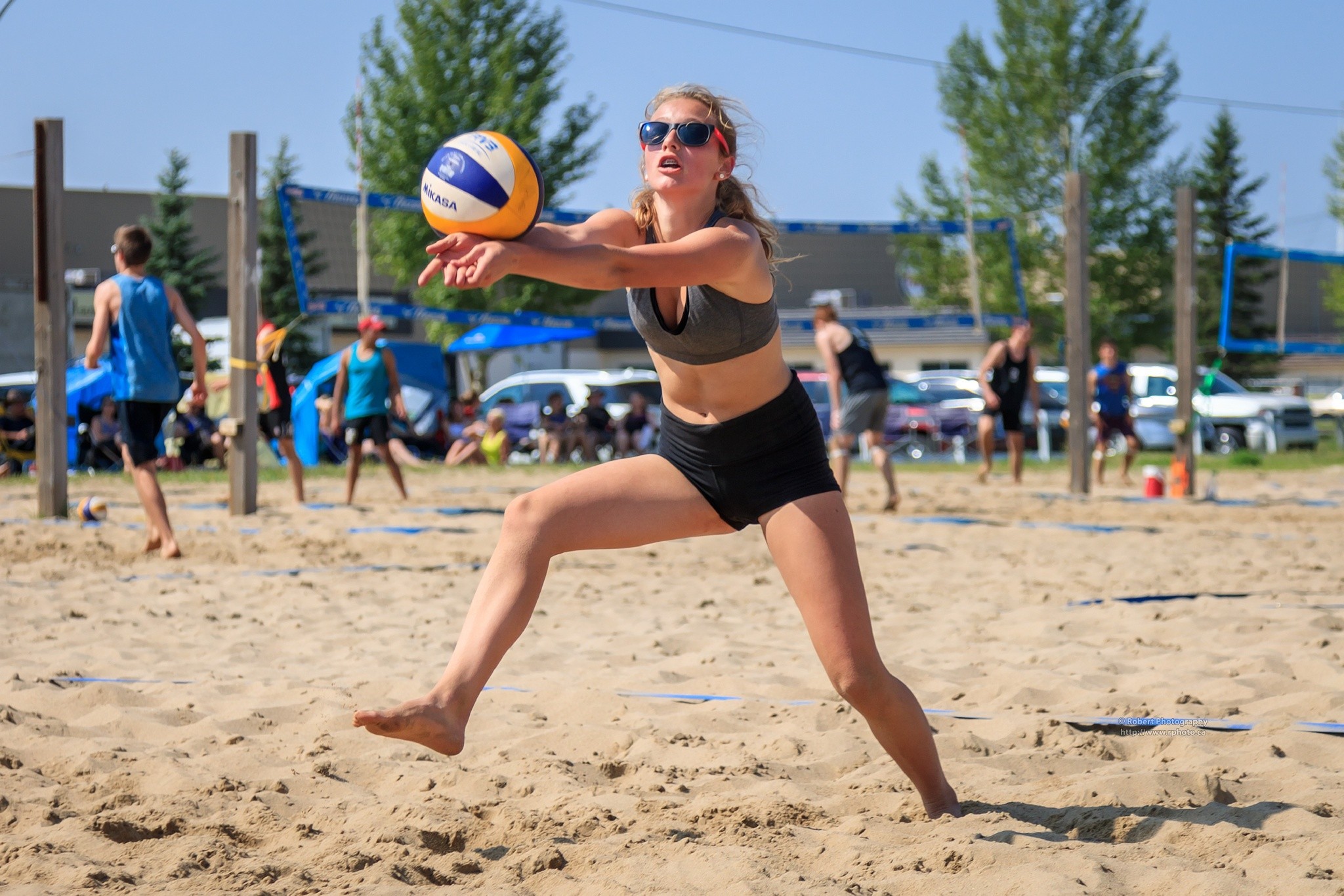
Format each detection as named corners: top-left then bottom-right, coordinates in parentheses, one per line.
top-left (1064, 67), bottom-right (1170, 495)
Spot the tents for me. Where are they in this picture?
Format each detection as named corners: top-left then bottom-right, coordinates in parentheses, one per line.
top-left (163, 368), bottom-right (255, 470)
top-left (28, 351), bottom-right (179, 473)
top-left (292, 338), bottom-right (454, 467)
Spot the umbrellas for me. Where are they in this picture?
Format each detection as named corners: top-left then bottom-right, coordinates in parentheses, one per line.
top-left (445, 320), bottom-right (596, 374)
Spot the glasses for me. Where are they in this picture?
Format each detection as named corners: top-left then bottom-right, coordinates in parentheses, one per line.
top-left (638, 120), bottom-right (731, 155)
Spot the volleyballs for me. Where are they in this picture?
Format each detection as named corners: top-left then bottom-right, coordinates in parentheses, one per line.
top-left (420, 131), bottom-right (544, 240)
top-left (77, 496), bottom-right (107, 521)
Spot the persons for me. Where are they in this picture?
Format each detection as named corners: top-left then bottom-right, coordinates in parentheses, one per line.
top-left (352, 85), bottom-right (959, 821)
top-left (1086, 337), bottom-right (1140, 492)
top-left (810, 302), bottom-right (900, 512)
top-left (84, 222), bottom-right (209, 560)
top-left (254, 313), bottom-right (306, 506)
top-left (329, 311), bottom-right (415, 504)
top-left (0, 385), bottom-right (660, 481)
top-left (970, 319), bottom-right (1042, 489)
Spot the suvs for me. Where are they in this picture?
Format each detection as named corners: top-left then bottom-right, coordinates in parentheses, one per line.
top-left (476, 367), bottom-right (669, 445)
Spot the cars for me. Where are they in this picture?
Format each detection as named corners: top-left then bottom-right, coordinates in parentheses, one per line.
top-left (1059, 363), bottom-right (1344, 454)
top-left (789, 369), bottom-right (1069, 452)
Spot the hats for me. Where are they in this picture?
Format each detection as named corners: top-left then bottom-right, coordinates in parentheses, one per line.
top-left (357, 316), bottom-right (386, 334)
top-left (804, 289), bottom-right (836, 306)
